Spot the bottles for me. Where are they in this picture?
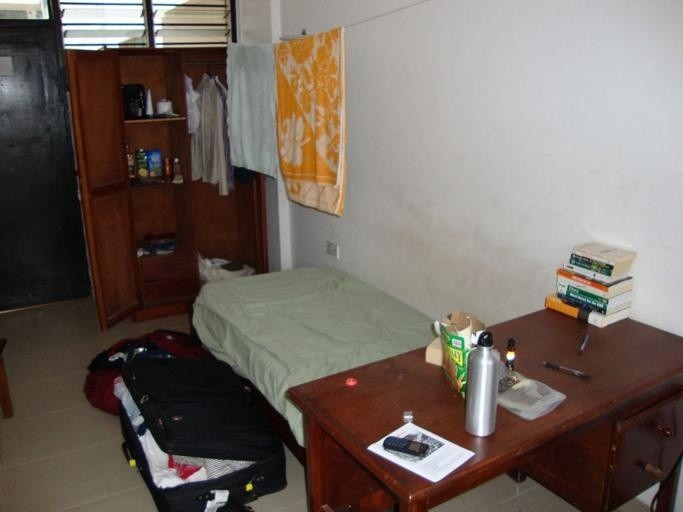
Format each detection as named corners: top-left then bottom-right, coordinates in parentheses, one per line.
top-left (136, 145), bottom-right (147, 176)
top-left (172, 157), bottom-right (183, 184)
top-left (137, 87), bottom-right (152, 119)
top-left (465, 331), bottom-right (497, 435)
top-left (125, 138), bottom-right (134, 178)
top-left (162, 157), bottom-right (171, 183)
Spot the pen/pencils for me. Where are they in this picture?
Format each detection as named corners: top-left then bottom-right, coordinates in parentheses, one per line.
top-left (579, 334), bottom-right (590, 352)
top-left (542, 361), bottom-right (592, 380)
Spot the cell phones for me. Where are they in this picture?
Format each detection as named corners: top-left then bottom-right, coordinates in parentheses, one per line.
top-left (383, 436), bottom-right (429, 456)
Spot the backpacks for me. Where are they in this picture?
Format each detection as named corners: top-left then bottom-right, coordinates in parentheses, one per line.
top-left (83, 328), bottom-right (210, 418)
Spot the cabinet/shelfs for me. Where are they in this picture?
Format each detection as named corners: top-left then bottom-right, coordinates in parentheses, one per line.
top-left (66, 48), bottom-right (269, 333)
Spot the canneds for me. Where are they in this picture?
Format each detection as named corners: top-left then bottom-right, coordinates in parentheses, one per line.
top-left (163, 158), bottom-right (172, 177)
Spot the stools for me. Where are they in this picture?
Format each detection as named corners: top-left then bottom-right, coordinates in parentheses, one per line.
top-left (0, 337), bottom-right (14, 418)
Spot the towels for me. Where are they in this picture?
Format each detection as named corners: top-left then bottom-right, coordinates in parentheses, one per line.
top-left (275, 25), bottom-right (347, 218)
top-left (226, 41), bottom-right (279, 180)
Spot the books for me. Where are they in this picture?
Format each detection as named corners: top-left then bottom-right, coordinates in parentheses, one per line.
top-left (366, 421), bottom-right (476, 482)
top-left (545, 238), bottom-right (637, 329)
top-left (495, 358), bottom-right (567, 421)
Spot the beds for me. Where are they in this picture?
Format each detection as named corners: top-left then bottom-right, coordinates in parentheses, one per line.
top-left (192, 265), bottom-right (438, 469)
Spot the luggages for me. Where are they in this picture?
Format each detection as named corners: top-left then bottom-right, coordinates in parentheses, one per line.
top-left (118, 341), bottom-right (290, 512)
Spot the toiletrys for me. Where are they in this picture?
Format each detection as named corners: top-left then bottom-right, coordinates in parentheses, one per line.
top-left (172, 158), bottom-right (180, 175)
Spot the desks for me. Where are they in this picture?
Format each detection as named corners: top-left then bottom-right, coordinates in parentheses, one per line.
top-left (287, 308), bottom-right (683, 512)
